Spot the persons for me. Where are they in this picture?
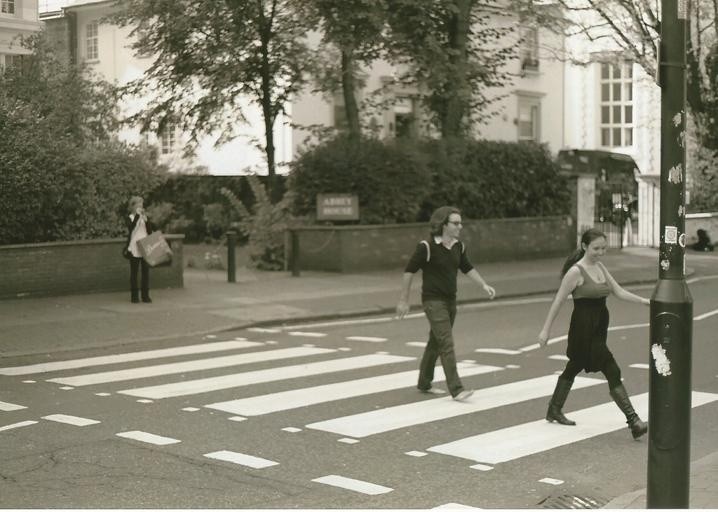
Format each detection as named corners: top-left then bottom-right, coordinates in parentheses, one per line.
top-left (396, 206), bottom-right (496, 400)
top-left (535, 229), bottom-right (651, 438)
top-left (125, 196), bottom-right (154, 302)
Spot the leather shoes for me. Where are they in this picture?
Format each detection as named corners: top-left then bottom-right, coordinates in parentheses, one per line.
top-left (131, 297), bottom-right (151, 303)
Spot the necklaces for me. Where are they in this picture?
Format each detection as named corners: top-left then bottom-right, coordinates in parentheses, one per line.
top-left (592, 267), bottom-right (606, 284)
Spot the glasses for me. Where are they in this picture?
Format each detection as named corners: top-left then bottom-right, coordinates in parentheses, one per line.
top-left (448, 220), bottom-right (459, 225)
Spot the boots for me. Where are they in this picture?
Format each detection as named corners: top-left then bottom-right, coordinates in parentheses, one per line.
top-left (610, 385), bottom-right (647, 438)
top-left (547, 377), bottom-right (576, 425)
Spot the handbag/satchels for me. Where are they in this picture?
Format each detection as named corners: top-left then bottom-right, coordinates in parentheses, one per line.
top-left (137, 231), bottom-right (172, 267)
top-left (123, 248), bottom-right (130, 258)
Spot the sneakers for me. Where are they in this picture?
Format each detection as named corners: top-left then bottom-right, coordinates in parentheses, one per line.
top-left (455, 389), bottom-right (473, 400)
top-left (423, 388), bottom-right (445, 394)
